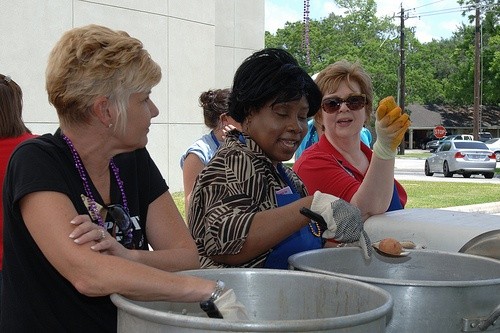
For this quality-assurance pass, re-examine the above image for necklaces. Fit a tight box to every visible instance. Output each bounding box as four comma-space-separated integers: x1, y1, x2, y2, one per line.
62, 133, 132, 250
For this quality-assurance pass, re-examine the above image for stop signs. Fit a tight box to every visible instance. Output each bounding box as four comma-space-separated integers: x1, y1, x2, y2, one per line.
432, 126, 447, 139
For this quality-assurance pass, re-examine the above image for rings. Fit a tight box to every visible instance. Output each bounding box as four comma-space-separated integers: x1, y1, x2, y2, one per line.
100, 228, 106, 238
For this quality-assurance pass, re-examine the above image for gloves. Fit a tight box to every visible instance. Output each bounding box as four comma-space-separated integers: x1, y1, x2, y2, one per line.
200, 287, 250, 321
310, 191, 372, 262
372, 104, 411, 160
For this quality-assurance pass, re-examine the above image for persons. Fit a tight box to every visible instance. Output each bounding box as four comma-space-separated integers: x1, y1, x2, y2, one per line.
0, 72, 41, 299
0, 24, 250, 332
186, 48, 371, 270
291, 59, 412, 249
180, 87, 232, 224
294, 71, 372, 163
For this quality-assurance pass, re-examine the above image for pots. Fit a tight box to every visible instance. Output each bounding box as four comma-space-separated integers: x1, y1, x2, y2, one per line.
289, 244, 500, 333
111, 268, 393, 333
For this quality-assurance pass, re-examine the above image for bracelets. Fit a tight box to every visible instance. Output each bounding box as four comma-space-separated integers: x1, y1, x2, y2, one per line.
210, 279, 225, 301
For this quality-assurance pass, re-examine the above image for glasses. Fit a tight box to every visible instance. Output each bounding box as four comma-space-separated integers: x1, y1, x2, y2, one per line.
320, 95, 369, 114
103, 204, 133, 250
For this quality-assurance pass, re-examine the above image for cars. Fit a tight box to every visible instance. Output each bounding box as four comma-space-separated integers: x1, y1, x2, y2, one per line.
425, 134, 473, 150
477, 132, 492, 141
424, 140, 497, 179
483, 138, 500, 163
